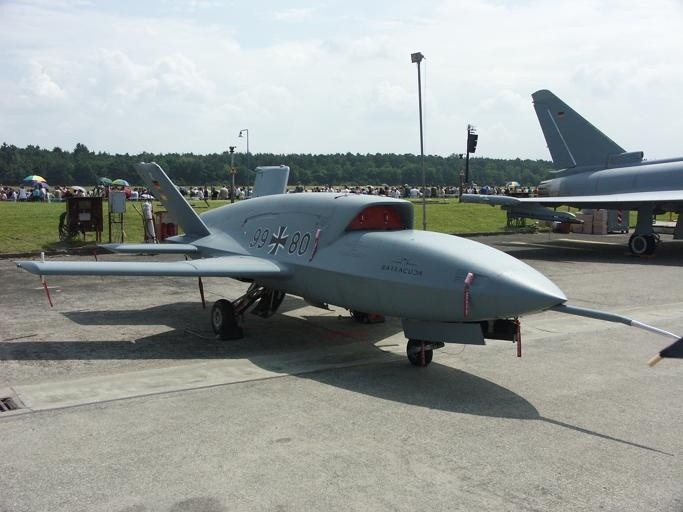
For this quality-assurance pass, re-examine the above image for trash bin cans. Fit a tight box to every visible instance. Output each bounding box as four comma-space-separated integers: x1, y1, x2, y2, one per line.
430, 187, 437, 197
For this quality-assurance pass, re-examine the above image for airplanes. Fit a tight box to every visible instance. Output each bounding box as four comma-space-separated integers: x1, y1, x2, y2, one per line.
460, 89, 683, 254
12, 160, 683, 367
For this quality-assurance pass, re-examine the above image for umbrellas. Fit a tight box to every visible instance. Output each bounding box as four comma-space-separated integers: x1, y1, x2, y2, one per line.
31, 182, 49, 187
506, 181, 520, 188
22, 175, 47, 183
97, 177, 112, 185
112, 179, 130, 187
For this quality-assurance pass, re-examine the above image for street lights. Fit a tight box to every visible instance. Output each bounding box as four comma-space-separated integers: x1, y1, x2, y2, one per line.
229, 146, 234, 201
411, 53, 427, 228
239, 129, 250, 196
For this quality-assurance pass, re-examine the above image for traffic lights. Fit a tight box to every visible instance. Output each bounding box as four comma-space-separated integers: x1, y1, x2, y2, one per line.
468, 133, 478, 153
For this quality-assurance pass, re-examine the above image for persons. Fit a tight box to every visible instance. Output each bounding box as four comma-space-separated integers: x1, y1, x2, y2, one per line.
175, 185, 252, 200
0, 184, 158, 202
285, 183, 539, 199
